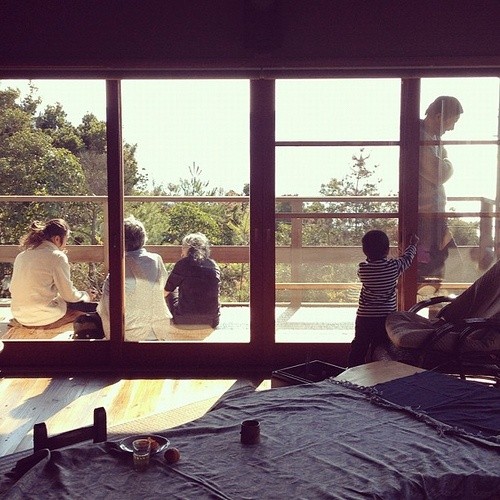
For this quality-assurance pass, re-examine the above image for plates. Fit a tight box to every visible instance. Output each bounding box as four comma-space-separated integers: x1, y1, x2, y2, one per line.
120, 435, 169, 454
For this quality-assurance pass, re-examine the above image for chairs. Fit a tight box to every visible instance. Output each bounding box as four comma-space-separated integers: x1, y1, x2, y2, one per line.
365, 259, 500, 376
33, 407, 107, 454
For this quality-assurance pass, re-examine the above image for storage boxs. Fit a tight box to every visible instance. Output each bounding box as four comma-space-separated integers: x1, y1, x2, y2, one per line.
271, 360, 346, 388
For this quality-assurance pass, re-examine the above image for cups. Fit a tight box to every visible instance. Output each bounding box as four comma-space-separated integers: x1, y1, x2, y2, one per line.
241, 419, 261, 445
133, 440, 150, 469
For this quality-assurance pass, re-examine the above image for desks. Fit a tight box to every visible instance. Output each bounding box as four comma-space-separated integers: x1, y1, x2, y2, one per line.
0, 360, 500, 500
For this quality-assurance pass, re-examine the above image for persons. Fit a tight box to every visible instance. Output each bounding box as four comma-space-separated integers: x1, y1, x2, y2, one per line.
96, 214, 173, 341
9, 219, 90, 329
347, 230, 420, 369
164, 233, 221, 330
417, 96, 463, 319
